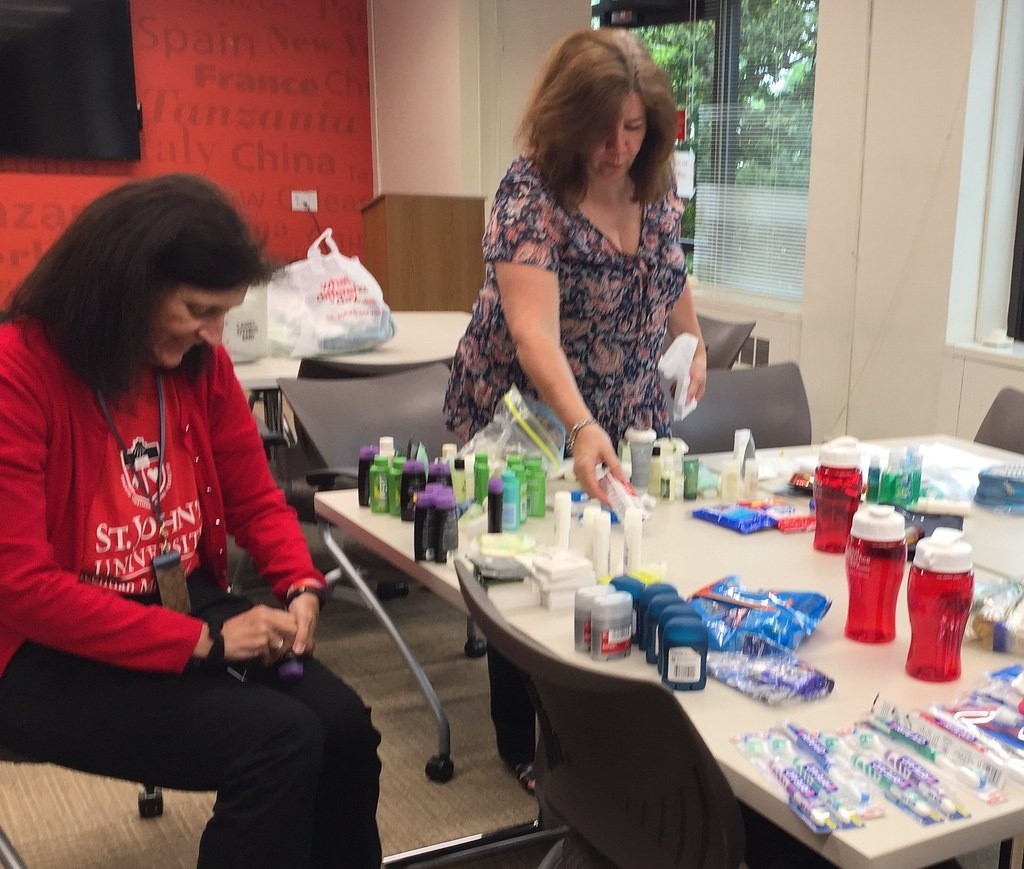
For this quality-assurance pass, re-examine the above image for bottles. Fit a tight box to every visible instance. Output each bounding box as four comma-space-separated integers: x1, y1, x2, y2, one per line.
844, 502, 907, 646
908, 528, 974, 683
814, 436, 862, 553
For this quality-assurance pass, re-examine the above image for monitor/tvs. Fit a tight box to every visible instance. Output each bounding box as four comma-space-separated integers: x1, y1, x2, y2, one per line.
0, 0, 142, 162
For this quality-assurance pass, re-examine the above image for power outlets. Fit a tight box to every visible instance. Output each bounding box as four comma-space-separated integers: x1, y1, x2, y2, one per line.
291, 190, 317, 212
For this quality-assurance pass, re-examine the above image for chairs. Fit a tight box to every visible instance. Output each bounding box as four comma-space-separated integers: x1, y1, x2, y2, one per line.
973, 387, 1024, 454
226, 356, 438, 608
660, 361, 812, 456
277, 361, 489, 783
453, 558, 962, 867
661, 312, 758, 372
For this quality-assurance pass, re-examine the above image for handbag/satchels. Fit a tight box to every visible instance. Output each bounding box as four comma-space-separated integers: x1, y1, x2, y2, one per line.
223, 228, 395, 364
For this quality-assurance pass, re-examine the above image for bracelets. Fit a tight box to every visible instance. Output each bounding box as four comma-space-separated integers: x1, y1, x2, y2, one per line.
284, 575, 327, 610
201, 619, 226, 673
564, 415, 600, 451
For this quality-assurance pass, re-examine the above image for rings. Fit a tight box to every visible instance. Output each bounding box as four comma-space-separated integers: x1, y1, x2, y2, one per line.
270, 634, 284, 653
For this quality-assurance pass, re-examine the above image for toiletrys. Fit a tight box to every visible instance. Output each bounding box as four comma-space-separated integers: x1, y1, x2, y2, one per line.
901, 527, 980, 682
611, 576, 643, 645
659, 616, 707, 692
589, 589, 634, 663
637, 582, 678, 652
353, 431, 922, 613
813, 435, 863, 554
643, 593, 685, 666
842, 502, 908, 645
657, 603, 703, 675
572, 584, 616, 653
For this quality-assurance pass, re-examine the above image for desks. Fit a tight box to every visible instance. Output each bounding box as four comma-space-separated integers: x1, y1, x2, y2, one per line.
313, 432, 1024, 869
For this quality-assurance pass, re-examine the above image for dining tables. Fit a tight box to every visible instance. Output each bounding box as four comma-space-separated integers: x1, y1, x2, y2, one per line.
298, 311, 475, 376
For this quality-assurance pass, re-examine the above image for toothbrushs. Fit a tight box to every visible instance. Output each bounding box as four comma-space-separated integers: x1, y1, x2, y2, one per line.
729, 689, 1020, 836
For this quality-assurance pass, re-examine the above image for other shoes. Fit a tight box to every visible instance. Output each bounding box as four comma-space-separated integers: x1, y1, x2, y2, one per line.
516, 763, 535, 793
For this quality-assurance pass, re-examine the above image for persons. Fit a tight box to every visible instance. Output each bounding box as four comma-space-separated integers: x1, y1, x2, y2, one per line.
443, 25, 708, 793
0, 170, 382, 869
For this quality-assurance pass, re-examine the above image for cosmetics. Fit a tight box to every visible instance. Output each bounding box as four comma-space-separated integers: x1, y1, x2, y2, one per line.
351, 425, 928, 589
